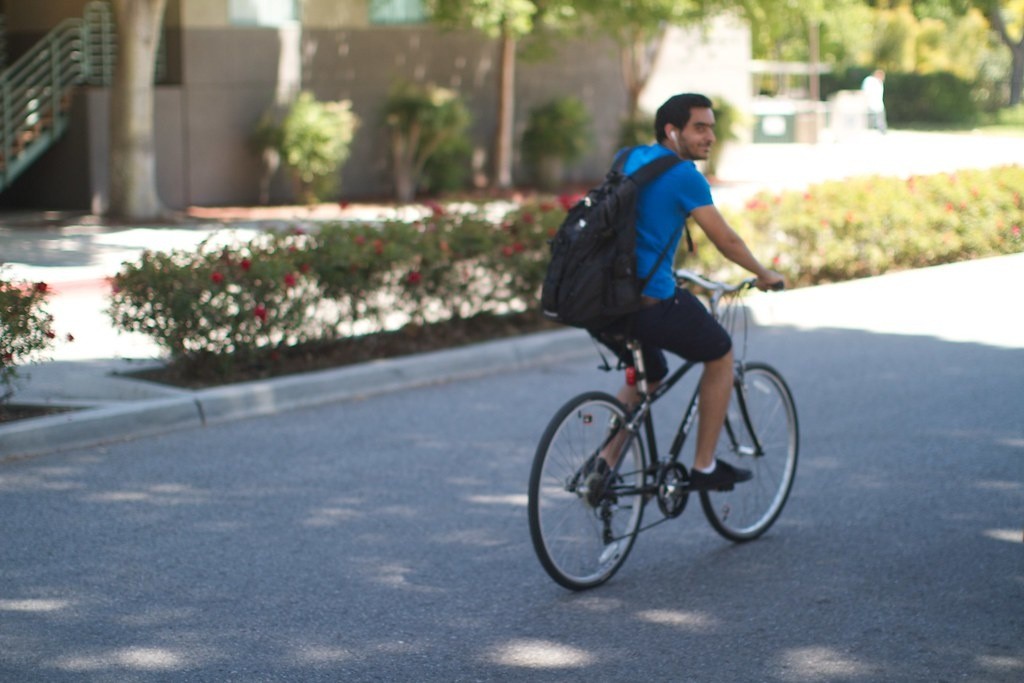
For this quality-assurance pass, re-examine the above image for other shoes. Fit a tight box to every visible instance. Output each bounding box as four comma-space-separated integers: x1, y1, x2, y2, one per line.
689, 458, 752, 489
594, 461, 618, 504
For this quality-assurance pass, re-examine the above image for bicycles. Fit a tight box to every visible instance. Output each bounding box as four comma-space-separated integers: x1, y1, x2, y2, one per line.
525, 264, 803, 594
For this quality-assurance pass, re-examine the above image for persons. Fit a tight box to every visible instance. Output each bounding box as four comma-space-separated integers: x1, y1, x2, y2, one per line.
586, 93, 781, 505
861, 70, 888, 135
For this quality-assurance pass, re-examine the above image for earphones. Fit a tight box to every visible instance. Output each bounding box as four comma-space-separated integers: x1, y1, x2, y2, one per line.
670, 130, 676, 144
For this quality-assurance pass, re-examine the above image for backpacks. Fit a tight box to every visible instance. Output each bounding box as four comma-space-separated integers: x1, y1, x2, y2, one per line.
541, 146, 687, 325
